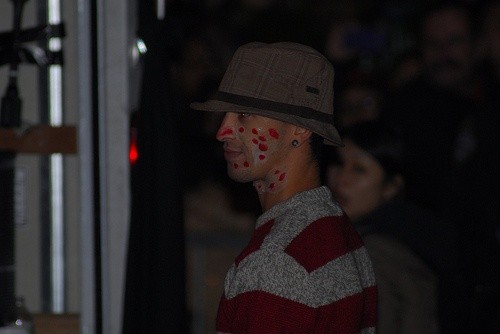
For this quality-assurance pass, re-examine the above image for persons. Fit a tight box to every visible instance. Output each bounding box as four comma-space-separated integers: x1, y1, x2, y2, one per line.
388, 0, 499, 216
327, 122, 444, 334
187, 43, 378, 333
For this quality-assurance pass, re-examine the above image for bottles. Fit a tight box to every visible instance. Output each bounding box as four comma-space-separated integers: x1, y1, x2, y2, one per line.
8, 298, 34, 334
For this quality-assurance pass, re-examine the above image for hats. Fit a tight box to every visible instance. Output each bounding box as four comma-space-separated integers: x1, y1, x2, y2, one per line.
196, 40, 347, 147
